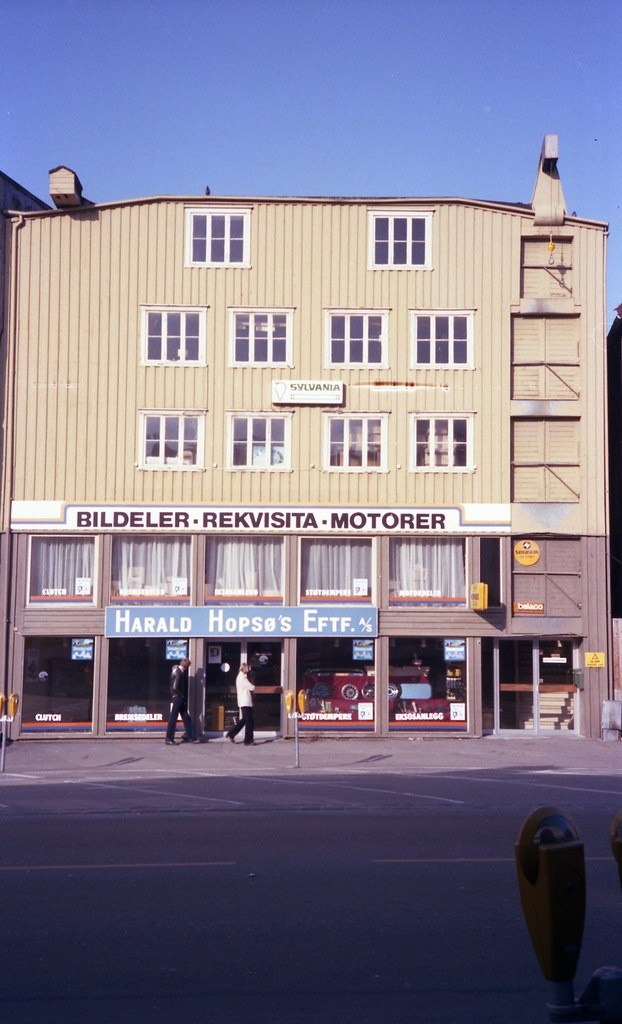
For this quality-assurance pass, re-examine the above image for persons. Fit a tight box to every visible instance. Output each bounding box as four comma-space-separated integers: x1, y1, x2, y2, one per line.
225, 663, 257, 746
165, 659, 201, 745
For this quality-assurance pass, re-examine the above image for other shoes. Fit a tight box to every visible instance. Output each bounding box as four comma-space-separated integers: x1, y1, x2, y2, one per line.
225, 734, 235, 744
166, 738, 177, 745
244, 741, 256, 746
190, 739, 200, 744
